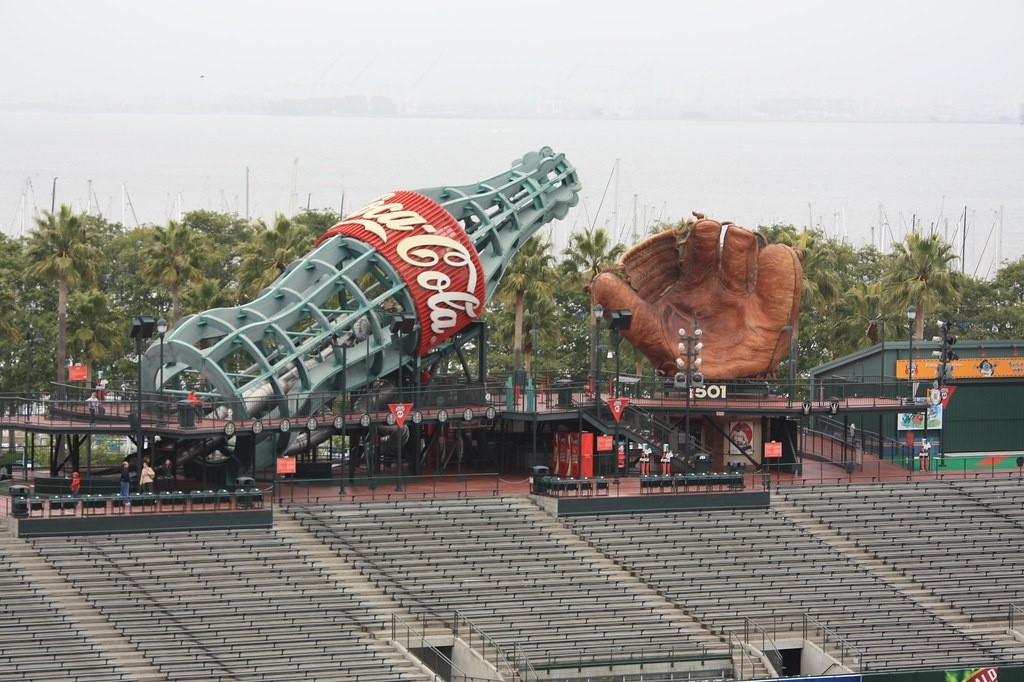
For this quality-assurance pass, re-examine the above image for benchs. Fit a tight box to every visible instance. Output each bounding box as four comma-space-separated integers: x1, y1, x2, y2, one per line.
0, 526, 414, 682
560, 506, 1024, 672
771, 476, 1024, 506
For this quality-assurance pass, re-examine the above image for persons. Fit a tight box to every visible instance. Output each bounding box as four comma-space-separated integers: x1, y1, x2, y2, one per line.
121, 456, 174, 505
70, 472, 81, 508
188, 390, 199, 416
85, 392, 100, 423
918, 433, 931, 472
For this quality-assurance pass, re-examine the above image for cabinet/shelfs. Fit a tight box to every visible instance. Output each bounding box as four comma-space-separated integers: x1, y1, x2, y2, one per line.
278, 489, 735, 681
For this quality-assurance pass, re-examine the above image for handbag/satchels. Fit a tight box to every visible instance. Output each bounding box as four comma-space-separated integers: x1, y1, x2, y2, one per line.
147, 467, 156, 478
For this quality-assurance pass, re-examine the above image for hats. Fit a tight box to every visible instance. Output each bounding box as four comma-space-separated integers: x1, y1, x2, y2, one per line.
73, 472, 78, 476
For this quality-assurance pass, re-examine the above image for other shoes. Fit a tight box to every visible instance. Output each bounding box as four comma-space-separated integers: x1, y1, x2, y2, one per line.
123, 501, 130, 505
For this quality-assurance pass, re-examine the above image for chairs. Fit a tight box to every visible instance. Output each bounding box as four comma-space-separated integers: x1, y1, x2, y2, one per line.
190, 490, 203, 512
639, 472, 747, 496
217, 489, 232, 512
159, 491, 173, 513
62, 494, 78, 516
30, 495, 45, 518
81, 494, 94, 516
235, 488, 249, 510
596, 476, 609, 496
172, 490, 188, 512
111, 493, 125, 514
540, 475, 593, 498
249, 488, 264, 509
129, 492, 143, 514
203, 489, 217, 511
142, 491, 157, 513
14, 497, 28, 518
49, 495, 63, 517
93, 494, 107, 515
